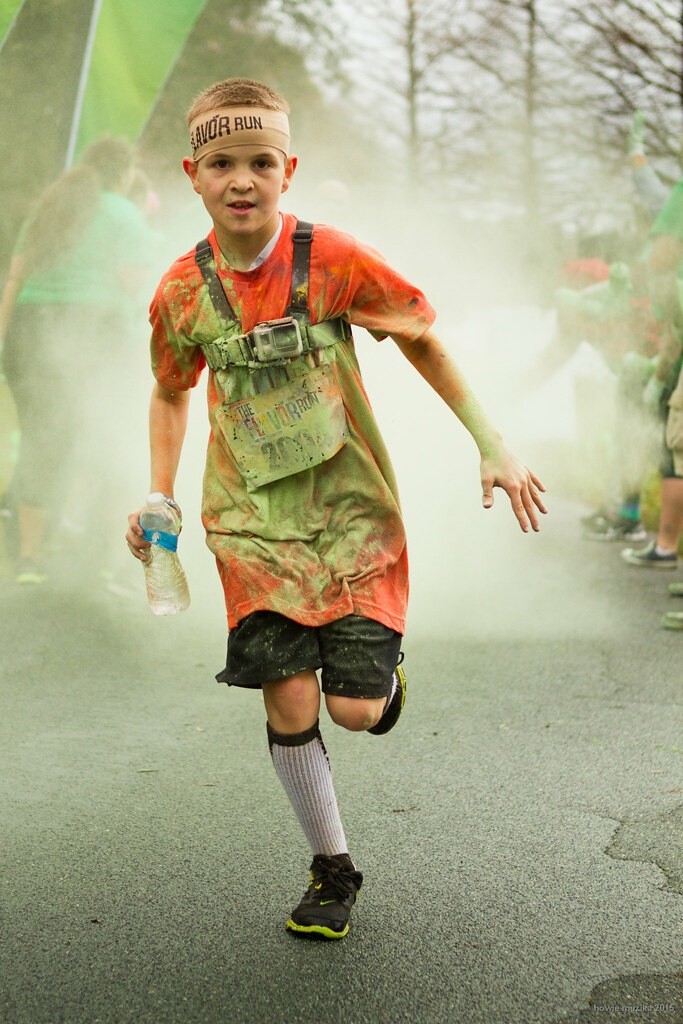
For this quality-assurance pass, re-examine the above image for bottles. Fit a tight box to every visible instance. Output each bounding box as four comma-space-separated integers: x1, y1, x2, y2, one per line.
138, 492, 191, 617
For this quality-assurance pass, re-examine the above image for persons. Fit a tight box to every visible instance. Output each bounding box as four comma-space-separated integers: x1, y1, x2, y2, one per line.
121, 78, 547, 940
0, 107, 683, 635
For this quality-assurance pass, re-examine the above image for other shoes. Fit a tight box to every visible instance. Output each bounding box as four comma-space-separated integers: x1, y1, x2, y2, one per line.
662, 610, 683, 628
588, 517, 647, 543
669, 583, 683, 595
14, 557, 47, 583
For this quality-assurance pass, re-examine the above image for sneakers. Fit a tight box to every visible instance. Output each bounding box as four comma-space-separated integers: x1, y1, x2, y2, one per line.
367, 663, 407, 736
285, 853, 364, 938
621, 540, 678, 568
